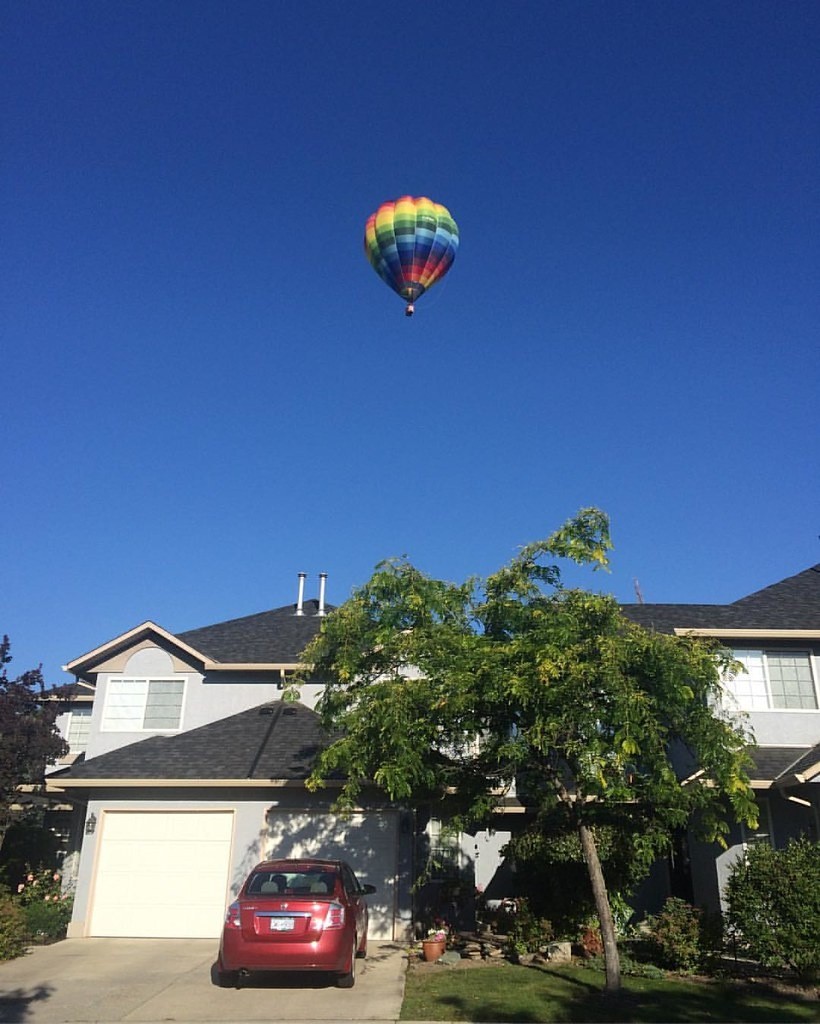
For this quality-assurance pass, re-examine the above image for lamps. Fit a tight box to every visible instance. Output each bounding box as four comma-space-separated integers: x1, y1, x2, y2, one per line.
86, 812, 96, 835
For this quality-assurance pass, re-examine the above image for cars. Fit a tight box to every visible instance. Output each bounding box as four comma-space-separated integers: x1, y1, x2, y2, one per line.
217, 856, 378, 988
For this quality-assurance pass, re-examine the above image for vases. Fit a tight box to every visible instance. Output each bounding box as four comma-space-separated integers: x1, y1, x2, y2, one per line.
422, 940, 445, 961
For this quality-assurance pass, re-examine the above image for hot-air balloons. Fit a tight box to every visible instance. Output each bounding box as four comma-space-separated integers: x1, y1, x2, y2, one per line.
364, 195, 461, 316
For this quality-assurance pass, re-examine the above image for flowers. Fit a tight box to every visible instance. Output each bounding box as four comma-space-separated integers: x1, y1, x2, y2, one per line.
426, 918, 447, 941
